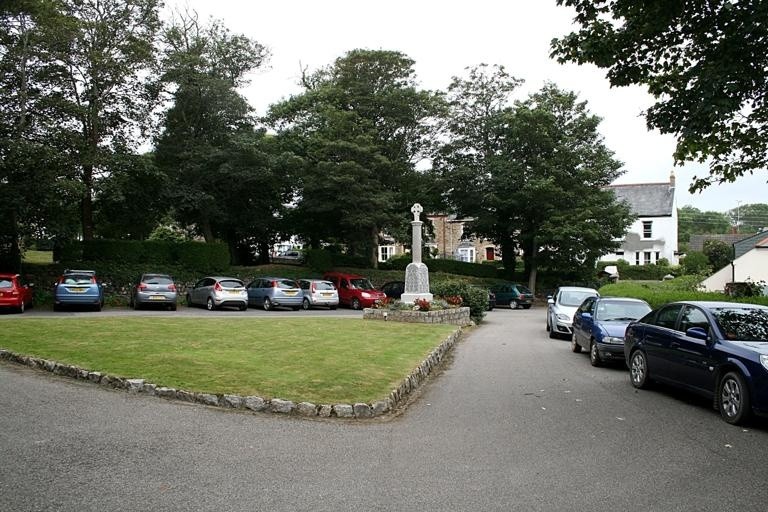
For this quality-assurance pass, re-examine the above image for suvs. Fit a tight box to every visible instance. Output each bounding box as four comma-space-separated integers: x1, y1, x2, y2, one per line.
322, 271, 387, 310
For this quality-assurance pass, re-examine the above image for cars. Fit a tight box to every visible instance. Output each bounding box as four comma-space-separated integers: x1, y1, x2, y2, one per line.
545, 285, 601, 340
186, 276, 248, 312
0, 273, 35, 313
130, 273, 178, 311
53, 267, 104, 312
624, 301, 768, 426
380, 281, 405, 299
246, 276, 340, 311
467, 283, 535, 311
275, 250, 305, 263
571, 296, 653, 368
